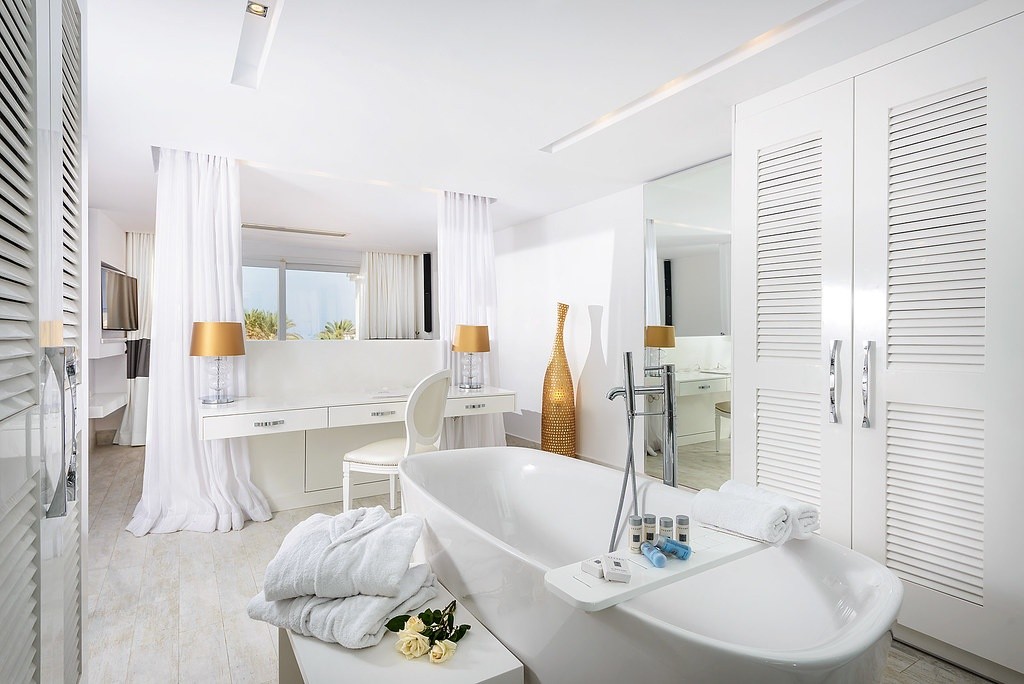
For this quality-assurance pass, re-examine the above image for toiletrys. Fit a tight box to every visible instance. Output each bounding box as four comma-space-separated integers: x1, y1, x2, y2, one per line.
628, 514, 691, 568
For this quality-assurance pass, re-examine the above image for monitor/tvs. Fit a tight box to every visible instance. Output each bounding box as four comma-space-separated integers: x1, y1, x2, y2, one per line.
100, 267, 139, 331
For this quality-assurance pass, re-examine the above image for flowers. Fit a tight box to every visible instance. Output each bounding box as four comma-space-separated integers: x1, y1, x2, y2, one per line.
384, 600, 471, 663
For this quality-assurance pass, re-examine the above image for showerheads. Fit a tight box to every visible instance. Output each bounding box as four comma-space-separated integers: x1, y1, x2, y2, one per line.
622, 351, 637, 419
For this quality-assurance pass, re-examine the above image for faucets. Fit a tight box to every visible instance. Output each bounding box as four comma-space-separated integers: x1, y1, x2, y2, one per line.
605, 363, 676, 402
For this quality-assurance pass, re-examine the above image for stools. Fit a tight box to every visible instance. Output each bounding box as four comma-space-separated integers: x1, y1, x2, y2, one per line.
278, 563, 524, 684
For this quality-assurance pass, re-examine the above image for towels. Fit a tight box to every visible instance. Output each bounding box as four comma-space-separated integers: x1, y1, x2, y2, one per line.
690, 479, 821, 549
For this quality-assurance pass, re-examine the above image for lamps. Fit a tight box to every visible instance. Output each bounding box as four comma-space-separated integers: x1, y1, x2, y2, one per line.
190, 322, 246, 405
451, 324, 490, 389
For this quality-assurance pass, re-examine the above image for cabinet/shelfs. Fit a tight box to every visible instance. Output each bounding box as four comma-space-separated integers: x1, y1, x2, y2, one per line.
730, 0, 1024, 684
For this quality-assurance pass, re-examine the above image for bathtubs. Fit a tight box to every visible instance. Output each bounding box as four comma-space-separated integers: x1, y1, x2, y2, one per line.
397, 444, 906, 683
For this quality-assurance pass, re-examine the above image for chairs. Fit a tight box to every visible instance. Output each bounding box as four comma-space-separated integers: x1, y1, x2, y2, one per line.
714, 401, 731, 453
343, 369, 452, 512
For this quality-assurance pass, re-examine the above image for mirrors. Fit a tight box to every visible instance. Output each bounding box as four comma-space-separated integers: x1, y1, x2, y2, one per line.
642, 153, 733, 493
101, 267, 139, 331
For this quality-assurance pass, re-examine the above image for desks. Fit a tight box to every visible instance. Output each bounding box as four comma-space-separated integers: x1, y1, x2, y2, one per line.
643, 366, 732, 456
197, 385, 517, 441
